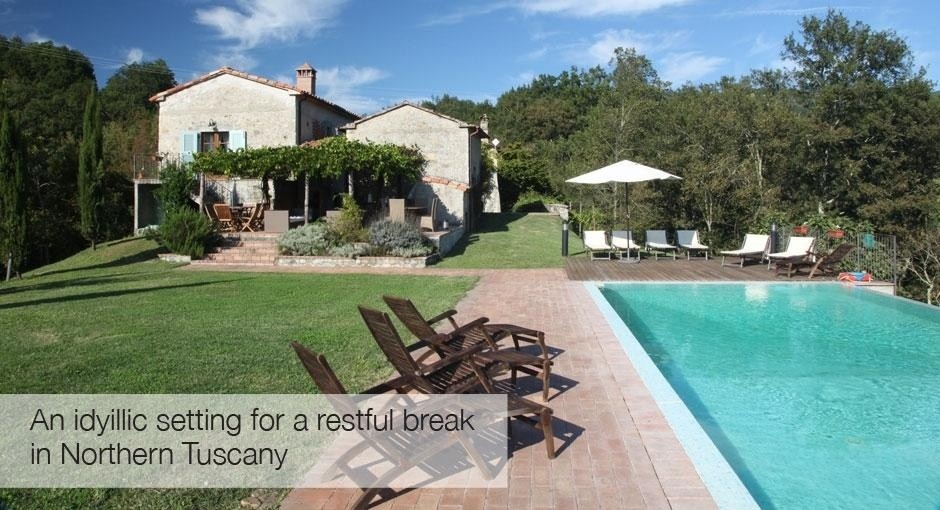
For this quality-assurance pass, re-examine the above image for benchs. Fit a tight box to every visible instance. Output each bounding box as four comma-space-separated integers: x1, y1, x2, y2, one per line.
644, 230, 676, 260
583, 231, 612, 261
805, 244, 855, 279
721, 234, 773, 269
612, 231, 640, 262
358, 304, 556, 405
383, 294, 548, 360
767, 236, 816, 278
676, 230, 709, 260
292, 340, 556, 510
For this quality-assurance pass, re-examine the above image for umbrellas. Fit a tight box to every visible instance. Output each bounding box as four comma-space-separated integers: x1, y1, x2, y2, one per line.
565, 160, 683, 259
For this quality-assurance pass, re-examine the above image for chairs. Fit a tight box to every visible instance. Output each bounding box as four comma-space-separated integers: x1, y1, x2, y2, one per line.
205, 201, 270, 232
